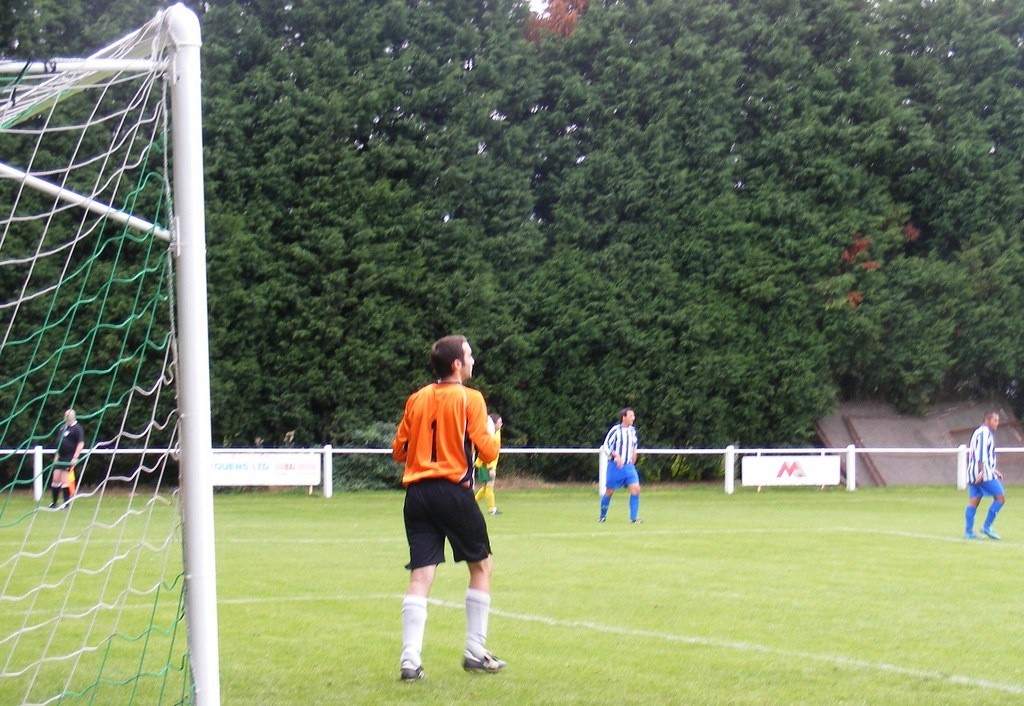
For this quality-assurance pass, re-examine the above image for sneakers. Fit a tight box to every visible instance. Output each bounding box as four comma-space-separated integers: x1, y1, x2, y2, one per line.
401, 661, 423, 682
463, 650, 506, 675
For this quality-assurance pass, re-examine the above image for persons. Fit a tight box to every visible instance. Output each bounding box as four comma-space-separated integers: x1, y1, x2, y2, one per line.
598, 407, 642, 524
48, 409, 86, 510
963, 411, 1005, 540
391, 335, 506, 679
472, 413, 504, 515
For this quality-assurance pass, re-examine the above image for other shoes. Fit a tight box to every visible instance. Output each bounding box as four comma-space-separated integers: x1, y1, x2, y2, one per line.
965, 532, 981, 539
599, 517, 606, 523
489, 509, 503, 514
629, 517, 642, 525
981, 526, 1000, 540
